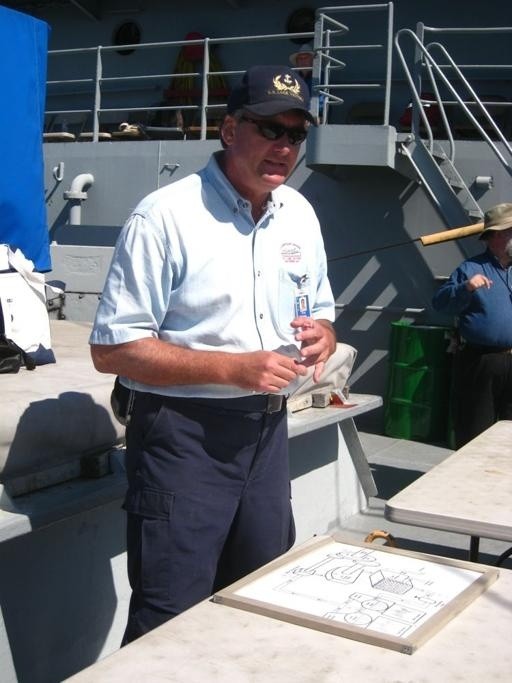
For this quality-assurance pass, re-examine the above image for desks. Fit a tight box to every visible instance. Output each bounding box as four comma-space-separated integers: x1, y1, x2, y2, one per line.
380, 420, 512, 535
52, 535, 512, 683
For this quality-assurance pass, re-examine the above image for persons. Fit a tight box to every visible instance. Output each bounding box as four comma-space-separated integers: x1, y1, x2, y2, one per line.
87, 62, 337, 649
431, 201, 512, 445
288, 41, 325, 126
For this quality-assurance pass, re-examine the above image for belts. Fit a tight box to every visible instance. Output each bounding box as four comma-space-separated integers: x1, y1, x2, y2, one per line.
190, 393, 288, 414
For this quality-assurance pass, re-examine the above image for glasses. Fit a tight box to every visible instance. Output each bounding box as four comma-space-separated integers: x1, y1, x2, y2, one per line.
237, 114, 308, 146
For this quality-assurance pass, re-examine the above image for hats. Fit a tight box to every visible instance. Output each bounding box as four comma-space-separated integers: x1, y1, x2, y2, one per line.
225, 64, 320, 130
477, 202, 512, 241
288, 44, 316, 67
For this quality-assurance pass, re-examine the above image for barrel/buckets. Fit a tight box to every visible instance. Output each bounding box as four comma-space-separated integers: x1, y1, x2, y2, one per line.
384, 326, 451, 440
